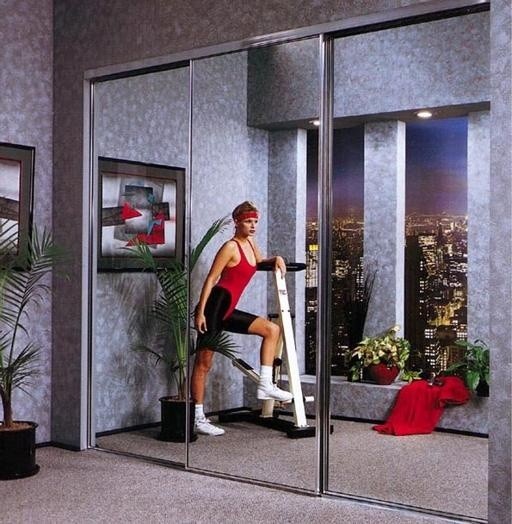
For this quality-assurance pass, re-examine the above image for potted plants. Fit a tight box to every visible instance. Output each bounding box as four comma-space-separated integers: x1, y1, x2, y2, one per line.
0, 211, 80, 480
113, 211, 272, 447
346, 321, 423, 387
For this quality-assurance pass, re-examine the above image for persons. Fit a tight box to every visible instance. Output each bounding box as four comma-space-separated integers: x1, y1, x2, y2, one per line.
189, 201, 294, 436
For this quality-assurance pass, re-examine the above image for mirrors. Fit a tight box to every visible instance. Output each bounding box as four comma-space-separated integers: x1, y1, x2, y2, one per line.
322, 6, 492, 524
186, 35, 321, 496
90, 64, 191, 476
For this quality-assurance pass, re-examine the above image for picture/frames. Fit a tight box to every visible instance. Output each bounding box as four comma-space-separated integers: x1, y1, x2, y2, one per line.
0, 141, 37, 273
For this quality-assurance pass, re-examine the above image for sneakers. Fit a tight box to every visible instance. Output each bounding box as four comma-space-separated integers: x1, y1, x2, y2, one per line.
193, 415, 225, 437
256, 382, 293, 402
